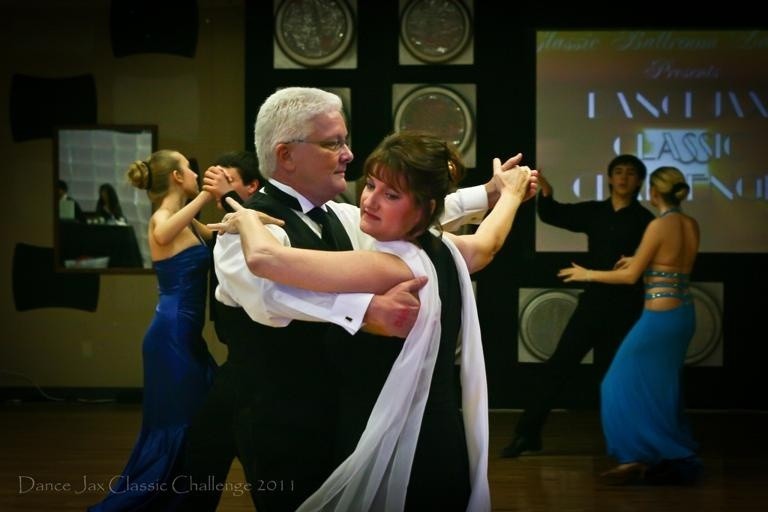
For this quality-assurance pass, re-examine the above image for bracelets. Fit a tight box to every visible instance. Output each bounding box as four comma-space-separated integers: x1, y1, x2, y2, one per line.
585, 268, 591, 282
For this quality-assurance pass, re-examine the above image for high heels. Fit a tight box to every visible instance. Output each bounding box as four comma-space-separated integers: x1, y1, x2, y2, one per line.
596, 458, 645, 482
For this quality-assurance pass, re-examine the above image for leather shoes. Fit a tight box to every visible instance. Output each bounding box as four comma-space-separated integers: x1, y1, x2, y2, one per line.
492, 432, 541, 459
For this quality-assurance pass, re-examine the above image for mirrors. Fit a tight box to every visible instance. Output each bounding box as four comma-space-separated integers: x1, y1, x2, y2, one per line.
49, 124, 160, 277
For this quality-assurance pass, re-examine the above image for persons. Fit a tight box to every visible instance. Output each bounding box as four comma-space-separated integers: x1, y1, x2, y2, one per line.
93, 182, 124, 223
204, 127, 531, 511
57, 179, 83, 219
499, 154, 657, 456
556, 166, 715, 488
127, 152, 264, 512
212, 82, 544, 512
85, 150, 233, 512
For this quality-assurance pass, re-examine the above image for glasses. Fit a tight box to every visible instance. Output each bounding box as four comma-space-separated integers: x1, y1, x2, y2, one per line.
286, 137, 350, 153
